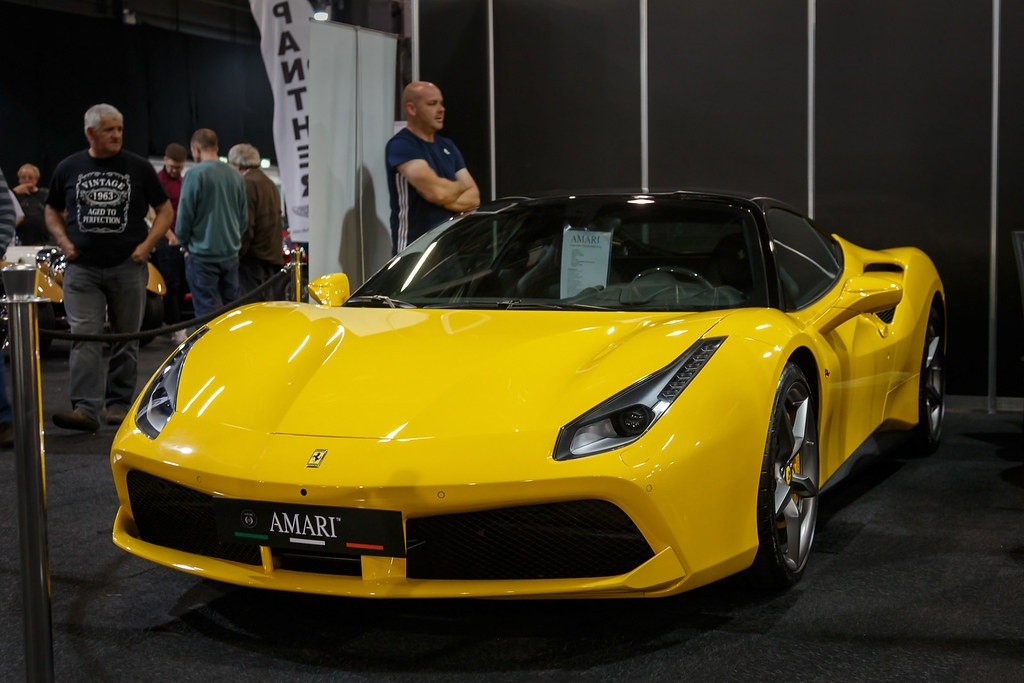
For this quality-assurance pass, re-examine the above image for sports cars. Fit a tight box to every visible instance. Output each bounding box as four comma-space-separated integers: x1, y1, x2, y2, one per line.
109, 189, 949, 599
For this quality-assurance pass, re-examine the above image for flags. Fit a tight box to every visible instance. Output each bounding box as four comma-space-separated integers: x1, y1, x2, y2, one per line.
249, 0, 317, 243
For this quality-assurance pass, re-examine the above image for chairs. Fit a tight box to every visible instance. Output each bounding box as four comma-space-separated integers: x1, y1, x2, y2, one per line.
702, 231, 800, 301
512, 226, 622, 298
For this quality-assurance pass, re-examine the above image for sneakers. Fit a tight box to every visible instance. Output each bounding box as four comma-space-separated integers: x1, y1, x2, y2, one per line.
105, 405, 128, 423
52, 408, 100, 432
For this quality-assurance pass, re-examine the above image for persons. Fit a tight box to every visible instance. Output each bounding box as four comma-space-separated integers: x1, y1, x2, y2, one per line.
229, 144, 284, 297
176, 129, 249, 321
44, 103, 174, 429
0, 168, 25, 267
12, 163, 50, 246
384, 81, 481, 255
150, 143, 187, 343
